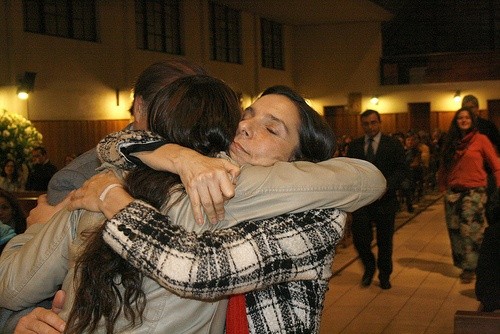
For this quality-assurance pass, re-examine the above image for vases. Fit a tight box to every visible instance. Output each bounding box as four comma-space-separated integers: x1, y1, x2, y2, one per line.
17, 162, 28, 189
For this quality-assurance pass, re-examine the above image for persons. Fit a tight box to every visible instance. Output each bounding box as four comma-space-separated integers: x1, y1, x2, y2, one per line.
345, 110, 407, 289
441, 95, 500, 313
389, 128, 448, 213
0, 60, 387, 334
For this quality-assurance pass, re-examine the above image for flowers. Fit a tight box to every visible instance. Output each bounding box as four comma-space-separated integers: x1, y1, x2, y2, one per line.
0, 108, 45, 174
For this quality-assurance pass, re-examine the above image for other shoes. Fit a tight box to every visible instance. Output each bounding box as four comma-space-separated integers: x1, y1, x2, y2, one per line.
361, 268, 376, 286
379, 276, 392, 290
460, 269, 475, 284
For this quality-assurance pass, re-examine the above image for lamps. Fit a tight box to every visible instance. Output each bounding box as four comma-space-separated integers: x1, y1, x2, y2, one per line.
370, 94, 379, 105
453, 89, 462, 101
17, 72, 38, 100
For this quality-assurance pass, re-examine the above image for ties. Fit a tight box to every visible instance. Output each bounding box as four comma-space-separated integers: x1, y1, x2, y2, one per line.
364, 139, 375, 162
